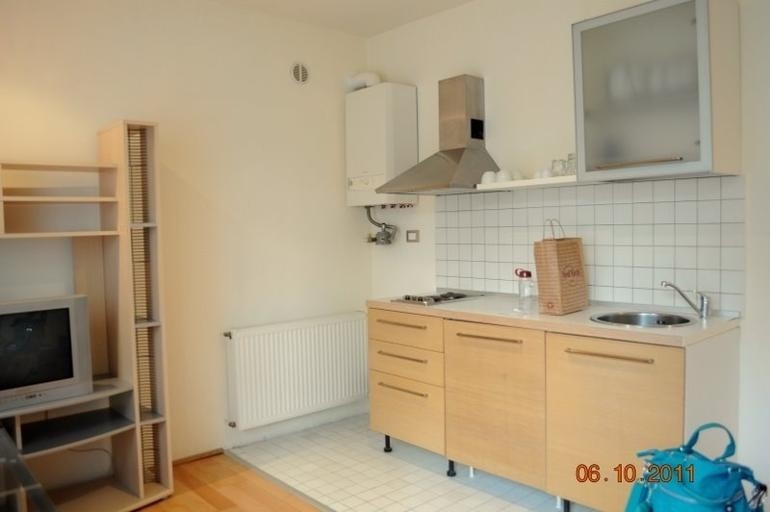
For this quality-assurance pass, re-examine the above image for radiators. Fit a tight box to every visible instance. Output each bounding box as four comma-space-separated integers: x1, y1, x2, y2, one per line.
225, 309, 367, 430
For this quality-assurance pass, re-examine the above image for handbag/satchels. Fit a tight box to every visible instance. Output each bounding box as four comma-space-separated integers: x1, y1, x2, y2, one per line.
624, 423, 767, 512
534, 219, 587, 315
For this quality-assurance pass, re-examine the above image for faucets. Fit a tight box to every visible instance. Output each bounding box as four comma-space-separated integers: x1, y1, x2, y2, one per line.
661, 280, 709, 321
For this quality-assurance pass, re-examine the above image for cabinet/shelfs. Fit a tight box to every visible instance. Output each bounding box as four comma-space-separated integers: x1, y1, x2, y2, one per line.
441, 307, 548, 495
572, 1, 739, 183
0, 120, 174, 512
548, 317, 740, 511
364, 302, 455, 476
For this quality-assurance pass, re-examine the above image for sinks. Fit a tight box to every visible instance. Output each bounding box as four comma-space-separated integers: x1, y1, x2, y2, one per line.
590, 312, 696, 330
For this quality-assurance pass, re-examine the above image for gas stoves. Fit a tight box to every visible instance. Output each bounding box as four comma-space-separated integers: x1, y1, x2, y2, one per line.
389, 290, 482, 306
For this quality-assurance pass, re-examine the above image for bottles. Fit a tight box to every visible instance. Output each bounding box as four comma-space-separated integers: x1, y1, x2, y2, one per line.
514, 267, 536, 313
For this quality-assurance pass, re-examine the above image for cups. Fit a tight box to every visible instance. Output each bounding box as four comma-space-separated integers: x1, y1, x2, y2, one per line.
476, 153, 577, 184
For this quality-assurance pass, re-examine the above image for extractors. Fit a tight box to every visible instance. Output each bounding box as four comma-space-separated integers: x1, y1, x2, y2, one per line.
375, 73, 513, 194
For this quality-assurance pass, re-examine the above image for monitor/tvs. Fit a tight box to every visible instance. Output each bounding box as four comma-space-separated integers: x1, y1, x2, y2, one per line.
0, 294, 94, 412
